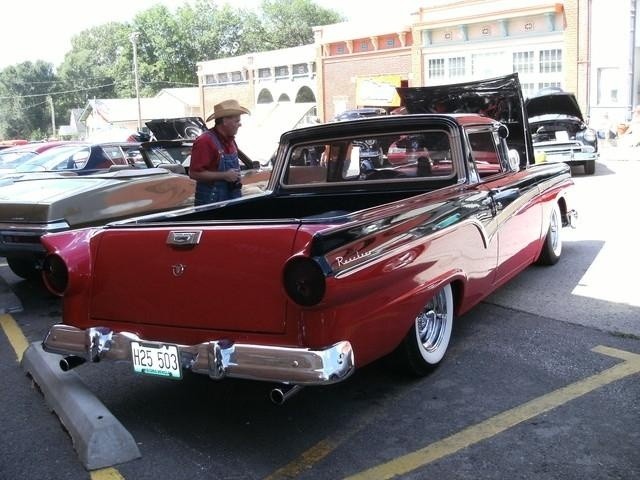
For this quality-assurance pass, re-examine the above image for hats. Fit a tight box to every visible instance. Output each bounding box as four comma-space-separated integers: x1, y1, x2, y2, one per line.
206, 101, 250, 122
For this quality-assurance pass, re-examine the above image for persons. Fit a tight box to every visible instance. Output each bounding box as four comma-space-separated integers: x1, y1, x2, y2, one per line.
307, 118, 325, 166
324, 119, 354, 177
188, 99, 251, 206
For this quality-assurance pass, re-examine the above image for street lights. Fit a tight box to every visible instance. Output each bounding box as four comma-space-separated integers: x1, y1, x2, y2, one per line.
127, 31, 142, 131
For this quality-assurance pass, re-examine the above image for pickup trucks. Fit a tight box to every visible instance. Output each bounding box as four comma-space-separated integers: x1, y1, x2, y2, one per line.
39, 70, 579, 407
